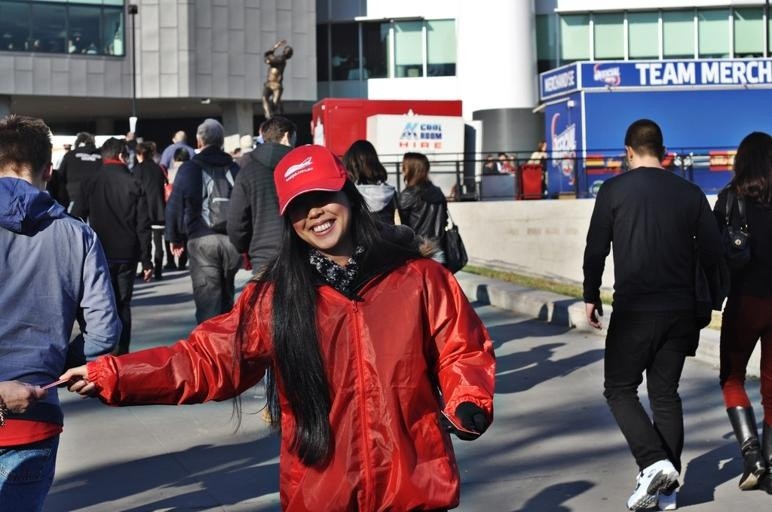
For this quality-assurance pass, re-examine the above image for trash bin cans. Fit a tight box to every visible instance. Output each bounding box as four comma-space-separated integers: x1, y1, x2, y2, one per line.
521, 164, 544, 200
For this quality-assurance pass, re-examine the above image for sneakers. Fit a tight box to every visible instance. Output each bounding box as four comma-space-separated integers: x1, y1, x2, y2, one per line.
626, 459, 679, 510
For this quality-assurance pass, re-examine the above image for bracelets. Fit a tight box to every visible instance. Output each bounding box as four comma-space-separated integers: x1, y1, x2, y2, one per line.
0, 396, 12, 427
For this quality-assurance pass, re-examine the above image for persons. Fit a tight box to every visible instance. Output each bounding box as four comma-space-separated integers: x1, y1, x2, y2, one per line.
57, 115, 296, 421
260, 44, 293, 121
340, 141, 400, 228
2, 31, 99, 56
483, 141, 551, 198
711, 131, 772, 492
0, 379, 50, 428
59, 144, 496, 512
397, 153, 450, 263
583, 118, 722, 510
0, 115, 122, 508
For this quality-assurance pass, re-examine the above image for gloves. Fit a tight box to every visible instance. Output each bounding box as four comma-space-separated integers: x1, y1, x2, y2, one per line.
438, 400, 489, 441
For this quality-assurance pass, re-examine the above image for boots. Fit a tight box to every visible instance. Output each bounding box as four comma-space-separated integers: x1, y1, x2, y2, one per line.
727, 405, 766, 489
758, 422, 772, 494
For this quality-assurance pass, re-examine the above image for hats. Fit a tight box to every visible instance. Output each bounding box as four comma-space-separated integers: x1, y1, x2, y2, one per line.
272, 144, 347, 216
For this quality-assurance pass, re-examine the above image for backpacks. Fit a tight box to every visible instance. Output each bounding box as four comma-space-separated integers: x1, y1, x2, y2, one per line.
193, 157, 236, 229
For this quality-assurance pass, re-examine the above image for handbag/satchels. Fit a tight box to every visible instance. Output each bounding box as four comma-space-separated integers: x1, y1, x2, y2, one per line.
164, 184, 173, 203
445, 224, 468, 273
724, 224, 752, 271
